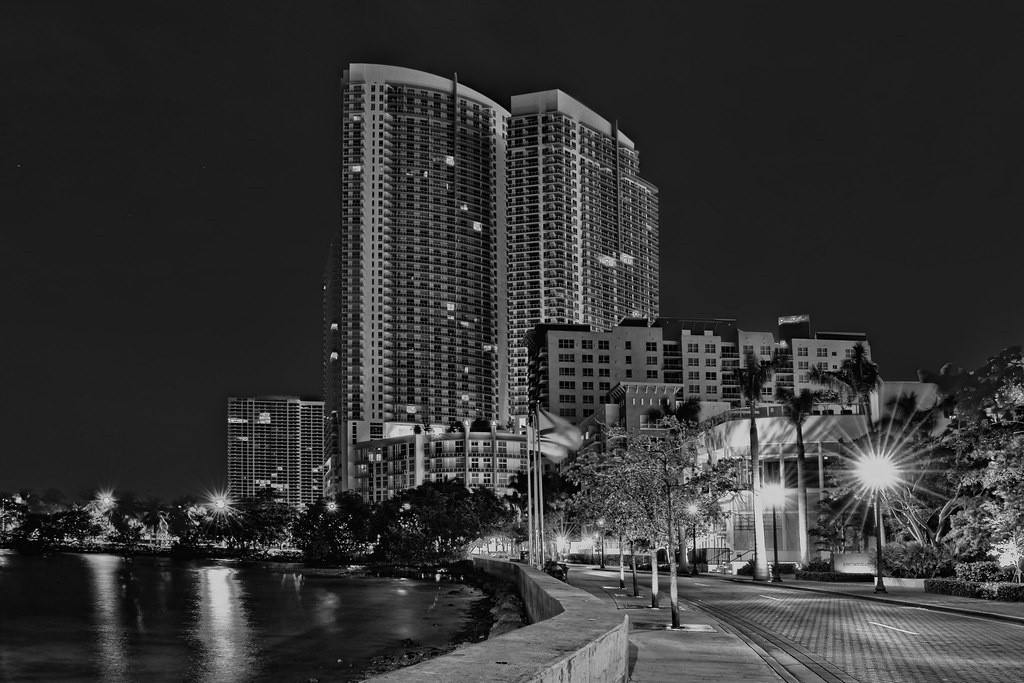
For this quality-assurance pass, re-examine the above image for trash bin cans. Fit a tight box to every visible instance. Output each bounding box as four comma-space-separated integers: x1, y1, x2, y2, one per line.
541, 560, 570, 584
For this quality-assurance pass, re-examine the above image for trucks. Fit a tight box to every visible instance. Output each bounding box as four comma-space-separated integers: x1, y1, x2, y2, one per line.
509, 551, 530, 564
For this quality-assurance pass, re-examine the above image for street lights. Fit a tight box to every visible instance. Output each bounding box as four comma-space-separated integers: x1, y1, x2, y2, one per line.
851, 449, 905, 595
597, 519, 607, 568
686, 505, 704, 577
759, 481, 792, 582
